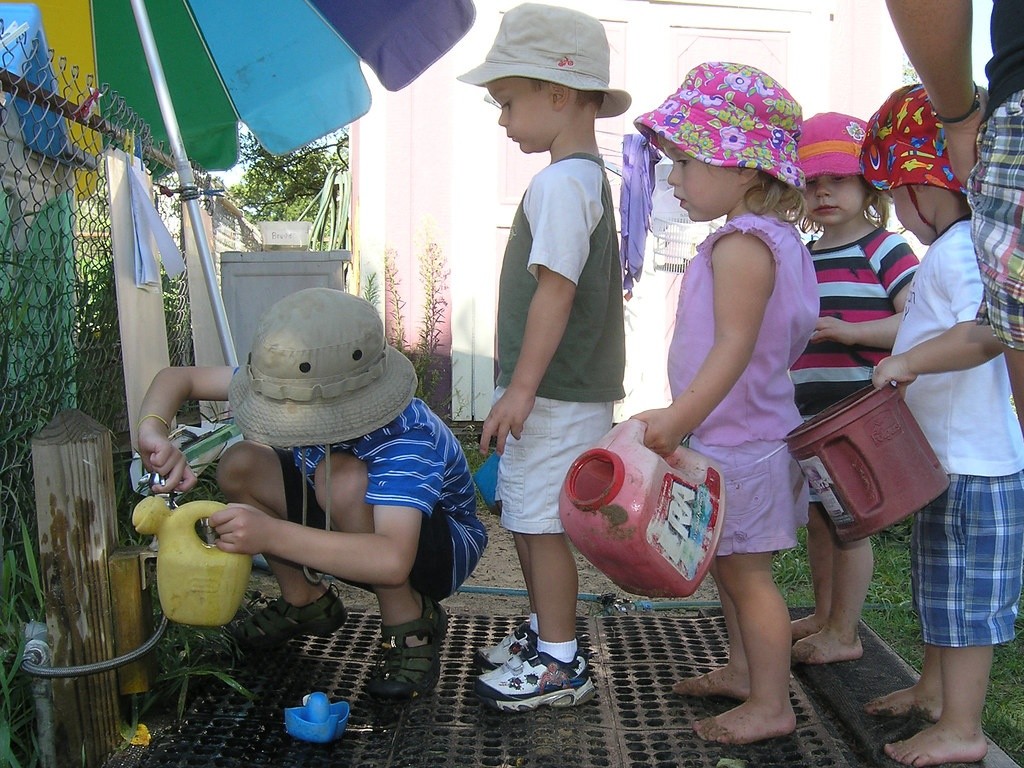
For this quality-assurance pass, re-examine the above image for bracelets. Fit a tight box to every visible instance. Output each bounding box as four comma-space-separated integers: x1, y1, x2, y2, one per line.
932, 80, 980, 123
138, 414, 171, 432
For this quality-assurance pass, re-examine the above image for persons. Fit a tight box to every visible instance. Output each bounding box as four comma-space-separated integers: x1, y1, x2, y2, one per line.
798, 112, 920, 663
458, 1, 632, 712
137, 288, 488, 698
629, 60, 821, 742
860, 0, 1024, 768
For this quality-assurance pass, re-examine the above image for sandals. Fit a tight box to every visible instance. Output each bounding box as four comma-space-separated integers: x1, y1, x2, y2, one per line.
366, 593, 449, 701
235, 586, 349, 653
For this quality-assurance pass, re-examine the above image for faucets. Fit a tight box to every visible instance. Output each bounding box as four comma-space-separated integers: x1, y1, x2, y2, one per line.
135, 468, 218, 551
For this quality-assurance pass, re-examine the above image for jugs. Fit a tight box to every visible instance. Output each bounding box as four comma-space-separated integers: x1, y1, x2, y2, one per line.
133, 496, 253, 627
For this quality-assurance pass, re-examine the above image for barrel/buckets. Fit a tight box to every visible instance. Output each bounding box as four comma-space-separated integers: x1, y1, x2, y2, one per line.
784, 380, 950, 542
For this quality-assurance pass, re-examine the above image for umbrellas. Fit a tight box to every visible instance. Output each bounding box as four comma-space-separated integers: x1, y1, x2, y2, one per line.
0, 0, 475, 367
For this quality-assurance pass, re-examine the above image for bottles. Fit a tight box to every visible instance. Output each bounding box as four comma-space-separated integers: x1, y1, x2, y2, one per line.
559, 420, 725, 599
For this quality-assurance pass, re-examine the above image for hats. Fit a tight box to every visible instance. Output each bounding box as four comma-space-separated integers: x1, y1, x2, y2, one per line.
226, 287, 418, 448
458, 2, 633, 118
630, 60, 806, 190
857, 83, 976, 194
789, 111, 872, 188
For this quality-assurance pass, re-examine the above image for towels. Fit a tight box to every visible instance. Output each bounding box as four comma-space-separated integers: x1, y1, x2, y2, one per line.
618, 132, 663, 293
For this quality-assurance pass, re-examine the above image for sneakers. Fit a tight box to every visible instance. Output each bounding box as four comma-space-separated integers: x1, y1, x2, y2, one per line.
475, 641, 597, 715
472, 621, 539, 675
